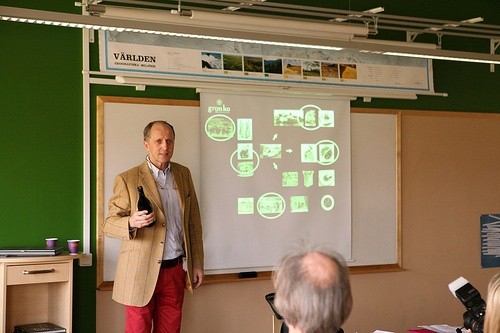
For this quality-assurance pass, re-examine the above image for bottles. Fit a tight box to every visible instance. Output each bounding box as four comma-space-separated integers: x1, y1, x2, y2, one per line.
136, 185, 156, 227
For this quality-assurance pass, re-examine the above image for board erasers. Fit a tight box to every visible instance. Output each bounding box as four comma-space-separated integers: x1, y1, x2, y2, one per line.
238, 271, 258, 278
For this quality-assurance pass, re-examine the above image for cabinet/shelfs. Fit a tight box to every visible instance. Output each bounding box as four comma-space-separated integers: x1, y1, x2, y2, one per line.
0, 251, 79, 333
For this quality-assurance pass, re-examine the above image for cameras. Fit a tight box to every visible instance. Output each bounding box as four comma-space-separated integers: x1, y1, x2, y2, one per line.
449, 276, 487, 333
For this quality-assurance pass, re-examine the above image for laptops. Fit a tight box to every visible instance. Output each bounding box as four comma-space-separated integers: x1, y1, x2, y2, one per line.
0, 246, 63, 256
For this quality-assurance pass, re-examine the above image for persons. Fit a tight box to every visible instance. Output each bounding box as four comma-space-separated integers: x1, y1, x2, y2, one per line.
102, 120, 206, 333
480, 271, 500, 333
271, 245, 353, 333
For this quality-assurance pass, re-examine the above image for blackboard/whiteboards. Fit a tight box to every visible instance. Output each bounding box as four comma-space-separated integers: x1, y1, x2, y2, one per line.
93, 93, 410, 292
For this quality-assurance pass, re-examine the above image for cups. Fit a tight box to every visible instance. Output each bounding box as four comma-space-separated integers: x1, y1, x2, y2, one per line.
45, 238, 59, 249
67, 239, 80, 255
